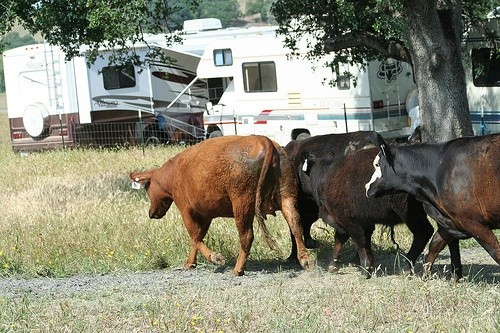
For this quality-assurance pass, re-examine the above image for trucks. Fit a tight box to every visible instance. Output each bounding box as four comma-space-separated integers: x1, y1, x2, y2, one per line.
197, 22, 419, 148
407, 7, 500, 142
2, 18, 294, 152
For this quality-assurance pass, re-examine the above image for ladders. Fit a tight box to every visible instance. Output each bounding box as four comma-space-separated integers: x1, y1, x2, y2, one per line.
384, 58, 401, 125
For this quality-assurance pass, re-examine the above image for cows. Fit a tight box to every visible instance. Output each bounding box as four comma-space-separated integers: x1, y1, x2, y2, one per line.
283, 125, 434, 280
129, 134, 315, 276
365, 125, 500, 283
